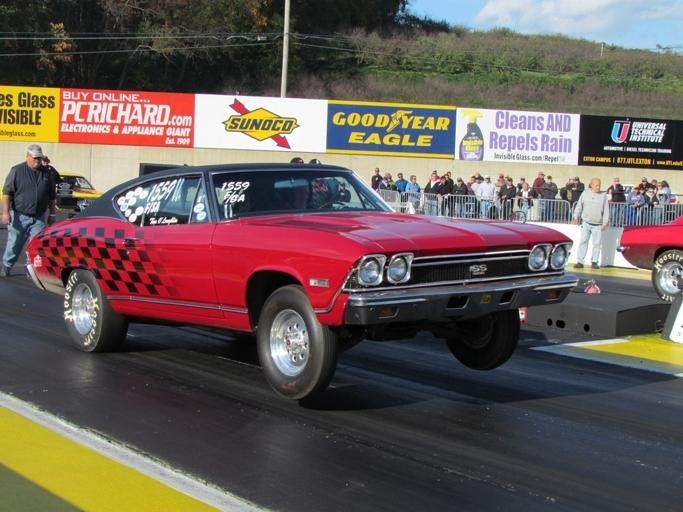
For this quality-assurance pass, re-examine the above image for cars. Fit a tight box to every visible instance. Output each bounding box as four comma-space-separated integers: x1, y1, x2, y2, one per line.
615, 215, 683, 302
601, 184, 678, 206
1, 171, 101, 211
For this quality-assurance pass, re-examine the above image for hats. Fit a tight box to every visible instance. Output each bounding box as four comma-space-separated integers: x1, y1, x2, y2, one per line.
27, 144, 43, 158
384, 170, 579, 182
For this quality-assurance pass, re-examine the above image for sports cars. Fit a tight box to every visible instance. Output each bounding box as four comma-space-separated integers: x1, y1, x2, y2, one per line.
22, 163, 580, 402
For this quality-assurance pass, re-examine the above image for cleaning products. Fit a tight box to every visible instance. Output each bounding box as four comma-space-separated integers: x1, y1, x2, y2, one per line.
458, 109, 485, 160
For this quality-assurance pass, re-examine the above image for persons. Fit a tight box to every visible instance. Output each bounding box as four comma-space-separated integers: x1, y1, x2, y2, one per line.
424, 172, 584, 222
608, 177, 672, 225
371, 169, 421, 214
0, 143, 57, 279
41, 154, 61, 225
570, 177, 610, 270
292, 158, 351, 208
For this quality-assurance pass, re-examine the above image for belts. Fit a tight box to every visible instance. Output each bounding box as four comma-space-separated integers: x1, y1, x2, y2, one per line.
585, 222, 600, 225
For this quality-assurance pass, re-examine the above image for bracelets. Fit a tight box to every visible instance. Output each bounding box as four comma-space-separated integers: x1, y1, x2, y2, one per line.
48, 213, 57, 218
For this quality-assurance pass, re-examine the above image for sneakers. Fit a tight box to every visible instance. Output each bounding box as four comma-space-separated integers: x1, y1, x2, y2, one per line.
574, 263, 584, 267
0, 265, 10, 278
592, 262, 599, 269
25, 273, 31, 279
